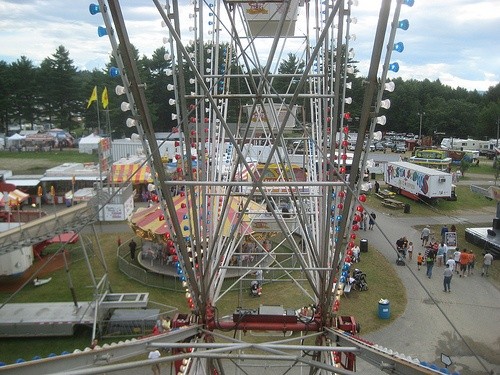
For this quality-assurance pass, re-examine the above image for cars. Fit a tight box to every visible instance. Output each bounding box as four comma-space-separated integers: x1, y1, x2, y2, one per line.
346, 139, 406, 154
24, 127, 75, 148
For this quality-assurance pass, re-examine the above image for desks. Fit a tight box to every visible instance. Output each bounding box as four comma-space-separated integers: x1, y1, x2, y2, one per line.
46, 196, 65, 203
384, 199, 403, 209
379, 191, 393, 198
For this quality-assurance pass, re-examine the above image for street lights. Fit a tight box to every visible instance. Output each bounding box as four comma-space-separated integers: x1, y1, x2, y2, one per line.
416, 111, 426, 146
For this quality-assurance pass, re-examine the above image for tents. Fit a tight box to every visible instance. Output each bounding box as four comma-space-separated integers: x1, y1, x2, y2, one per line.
79, 133, 103, 154
0, 189, 29, 207
8, 133, 25, 140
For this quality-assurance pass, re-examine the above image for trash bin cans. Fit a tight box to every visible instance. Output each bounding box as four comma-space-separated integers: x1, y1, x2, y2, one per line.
282, 205, 291, 218
404, 204, 410, 213
378, 301, 390, 319
266, 201, 275, 215
360, 238, 368, 252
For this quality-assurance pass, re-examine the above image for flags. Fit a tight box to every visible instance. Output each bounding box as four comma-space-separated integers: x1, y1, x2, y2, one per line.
86, 86, 97, 109
102, 87, 108, 109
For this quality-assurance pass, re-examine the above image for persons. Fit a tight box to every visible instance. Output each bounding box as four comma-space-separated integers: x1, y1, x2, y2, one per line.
456, 169, 461, 183
375, 181, 379, 193
396, 225, 493, 292
368, 182, 372, 191
129, 239, 137, 260
157, 315, 171, 333
148, 348, 161, 375
252, 269, 263, 284
359, 211, 376, 230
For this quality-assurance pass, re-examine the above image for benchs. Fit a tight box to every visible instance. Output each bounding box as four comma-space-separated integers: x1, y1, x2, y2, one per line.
382, 202, 405, 207
374, 192, 395, 198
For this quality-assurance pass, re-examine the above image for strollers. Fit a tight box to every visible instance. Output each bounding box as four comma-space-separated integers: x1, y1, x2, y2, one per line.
396, 246, 406, 266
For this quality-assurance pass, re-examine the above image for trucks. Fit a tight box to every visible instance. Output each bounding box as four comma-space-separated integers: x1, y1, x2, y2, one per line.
440, 137, 500, 157
